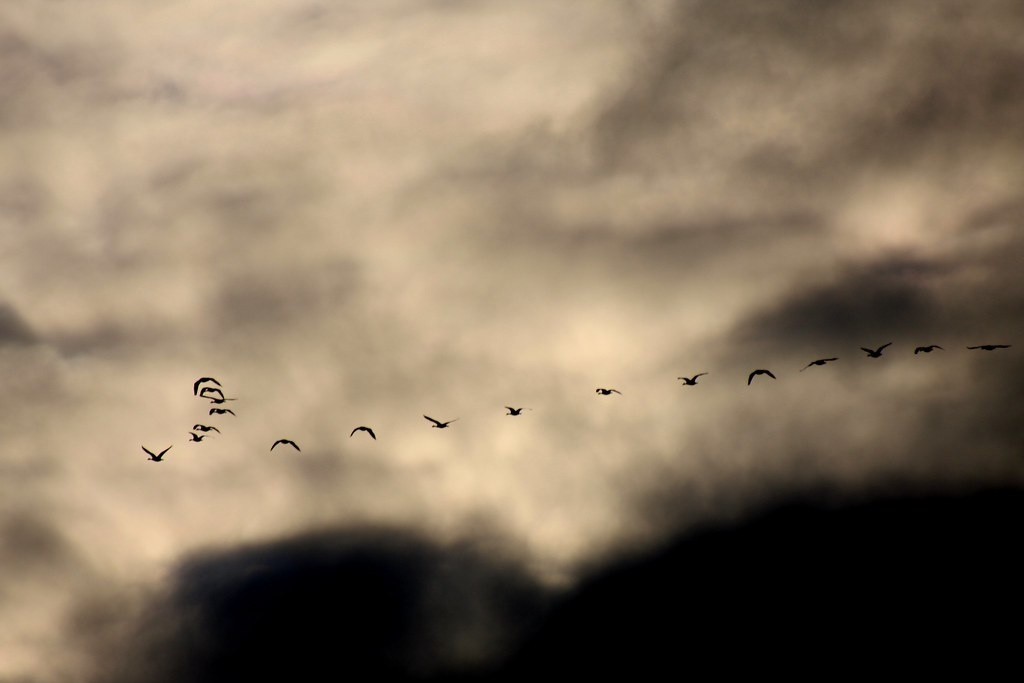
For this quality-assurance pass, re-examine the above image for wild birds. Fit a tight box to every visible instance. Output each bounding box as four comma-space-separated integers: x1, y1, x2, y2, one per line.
675, 372, 711, 386
269, 439, 303, 454
504, 404, 532, 417
422, 415, 458, 430
187, 376, 240, 442
746, 369, 777, 386
798, 357, 840, 373
859, 342, 893, 359
349, 426, 376, 441
965, 344, 1012, 352
913, 344, 945, 356
141, 444, 174, 463
595, 387, 625, 396
